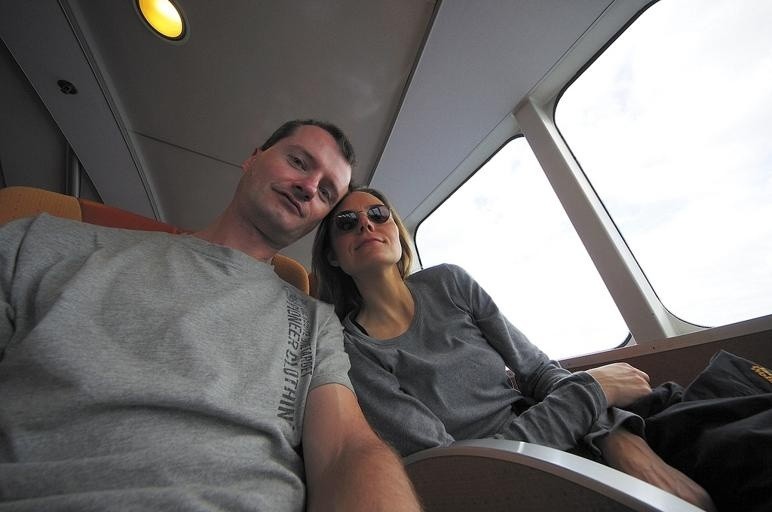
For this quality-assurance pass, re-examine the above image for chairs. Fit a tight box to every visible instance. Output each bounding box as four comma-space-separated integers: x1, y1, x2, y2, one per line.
1, 184, 309, 298
307, 270, 702, 511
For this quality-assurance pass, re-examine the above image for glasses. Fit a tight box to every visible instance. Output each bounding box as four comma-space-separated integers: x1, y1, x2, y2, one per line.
336, 205, 391, 231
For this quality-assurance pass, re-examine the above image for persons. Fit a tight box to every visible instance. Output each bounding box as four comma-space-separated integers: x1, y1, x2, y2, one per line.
312, 188, 772, 512
2, 119, 422, 510
